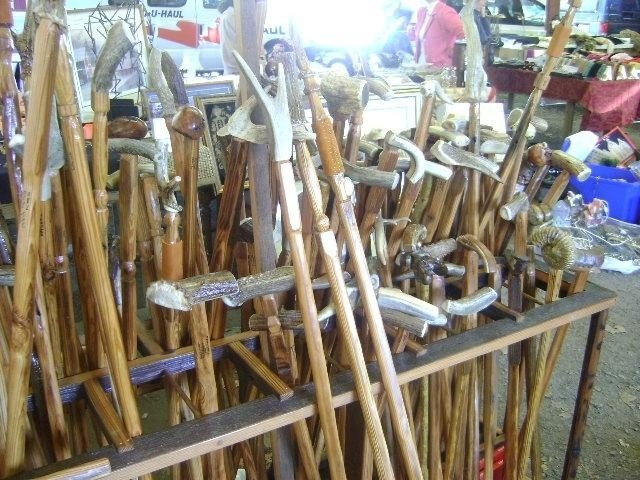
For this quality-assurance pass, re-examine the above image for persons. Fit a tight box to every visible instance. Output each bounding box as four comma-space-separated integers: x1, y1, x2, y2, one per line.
220, 0, 236, 76
473, 0, 492, 46
412, 1, 467, 88
263, 40, 294, 64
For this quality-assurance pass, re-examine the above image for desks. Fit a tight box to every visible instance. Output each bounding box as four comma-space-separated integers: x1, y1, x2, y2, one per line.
453, 65, 590, 139
